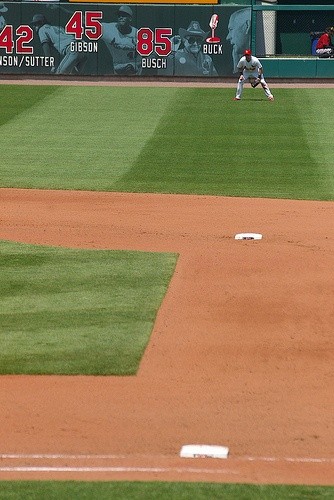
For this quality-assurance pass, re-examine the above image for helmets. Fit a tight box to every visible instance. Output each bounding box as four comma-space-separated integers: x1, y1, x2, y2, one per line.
244, 49, 251, 55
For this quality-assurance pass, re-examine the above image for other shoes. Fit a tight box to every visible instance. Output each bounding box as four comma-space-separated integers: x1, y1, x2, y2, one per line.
267, 95, 275, 101
233, 97, 242, 101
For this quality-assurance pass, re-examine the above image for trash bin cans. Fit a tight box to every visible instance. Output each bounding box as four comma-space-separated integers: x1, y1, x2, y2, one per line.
311, 32, 324, 56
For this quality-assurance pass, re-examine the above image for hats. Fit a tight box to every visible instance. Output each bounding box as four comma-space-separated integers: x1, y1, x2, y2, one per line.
30, 14, 47, 24
178, 21, 210, 41
116, 5, 133, 17
0, 2, 8, 13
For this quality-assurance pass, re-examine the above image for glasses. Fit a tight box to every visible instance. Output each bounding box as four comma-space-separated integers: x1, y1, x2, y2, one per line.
184, 37, 204, 46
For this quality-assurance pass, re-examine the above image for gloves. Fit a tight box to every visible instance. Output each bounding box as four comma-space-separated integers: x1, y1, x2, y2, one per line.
51, 67, 56, 73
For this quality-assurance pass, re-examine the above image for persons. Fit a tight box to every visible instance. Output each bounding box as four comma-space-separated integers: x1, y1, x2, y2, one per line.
165, 21, 217, 76
233, 49, 275, 102
315, 26, 334, 59
226, 6, 253, 74
46, 4, 147, 76
29, 14, 94, 76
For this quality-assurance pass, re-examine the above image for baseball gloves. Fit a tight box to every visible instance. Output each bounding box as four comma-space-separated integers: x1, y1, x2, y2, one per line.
251, 78, 260, 88
